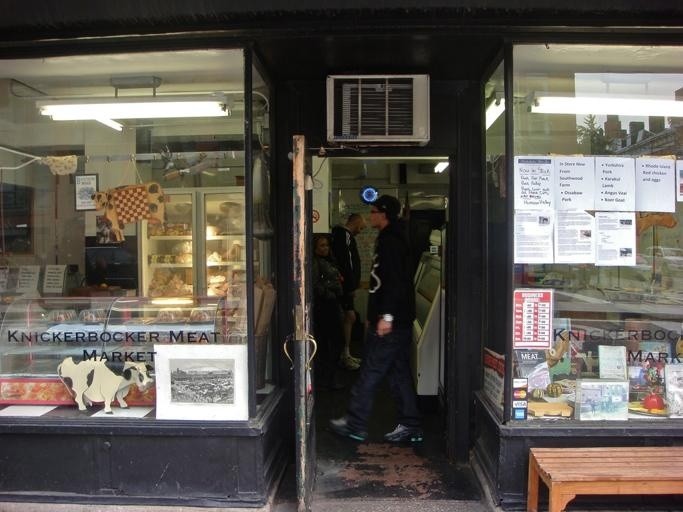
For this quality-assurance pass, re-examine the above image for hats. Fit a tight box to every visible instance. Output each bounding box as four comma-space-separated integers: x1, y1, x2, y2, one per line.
370, 195, 401, 215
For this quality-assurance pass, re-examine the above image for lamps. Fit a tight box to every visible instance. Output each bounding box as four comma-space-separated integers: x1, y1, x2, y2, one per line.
33, 74, 231, 123
523, 91, 681, 118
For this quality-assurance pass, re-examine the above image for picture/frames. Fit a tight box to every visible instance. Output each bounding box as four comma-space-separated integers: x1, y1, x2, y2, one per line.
73, 173, 98, 212
150, 342, 248, 422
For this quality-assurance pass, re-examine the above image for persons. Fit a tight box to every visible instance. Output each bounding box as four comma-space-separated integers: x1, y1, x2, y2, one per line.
309, 194, 425, 442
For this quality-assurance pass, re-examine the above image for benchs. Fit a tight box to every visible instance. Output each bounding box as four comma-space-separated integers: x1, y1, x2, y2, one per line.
527, 445, 683, 511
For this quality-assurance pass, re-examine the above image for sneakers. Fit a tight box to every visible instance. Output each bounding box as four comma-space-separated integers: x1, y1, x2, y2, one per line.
319, 382, 345, 393
384, 424, 423, 443
328, 417, 368, 441
338, 353, 363, 370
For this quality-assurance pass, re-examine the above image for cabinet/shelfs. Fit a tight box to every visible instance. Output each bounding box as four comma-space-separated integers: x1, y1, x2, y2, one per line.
0, 293, 225, 406
133, 185, 245, 318
